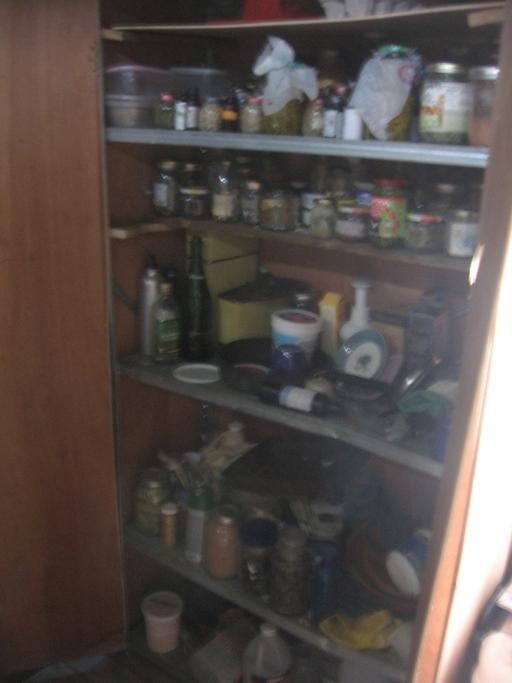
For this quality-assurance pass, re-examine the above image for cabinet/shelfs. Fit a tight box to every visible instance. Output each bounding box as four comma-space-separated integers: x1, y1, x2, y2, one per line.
95, 0, 512, 680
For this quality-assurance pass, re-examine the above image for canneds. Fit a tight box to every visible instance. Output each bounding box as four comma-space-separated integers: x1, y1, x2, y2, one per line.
205, 504, 277, 599
152, 62, 499, 261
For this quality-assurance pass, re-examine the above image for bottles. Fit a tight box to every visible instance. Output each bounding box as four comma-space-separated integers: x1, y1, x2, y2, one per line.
132, 473, 175, 537
418, 63, 500, 147
155, 85, 263, 134
180, 234, 218, 362
368, 177, 412, 250
204, 503, 243, 581
184, 486, 214, 564
405, 182, 481, 257
136, 254, 180, 364
152, 155, 370, 244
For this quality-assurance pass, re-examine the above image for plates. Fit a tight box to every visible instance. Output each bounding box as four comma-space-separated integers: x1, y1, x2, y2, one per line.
332, 328, 390, 380
172, 363, 224, 386
